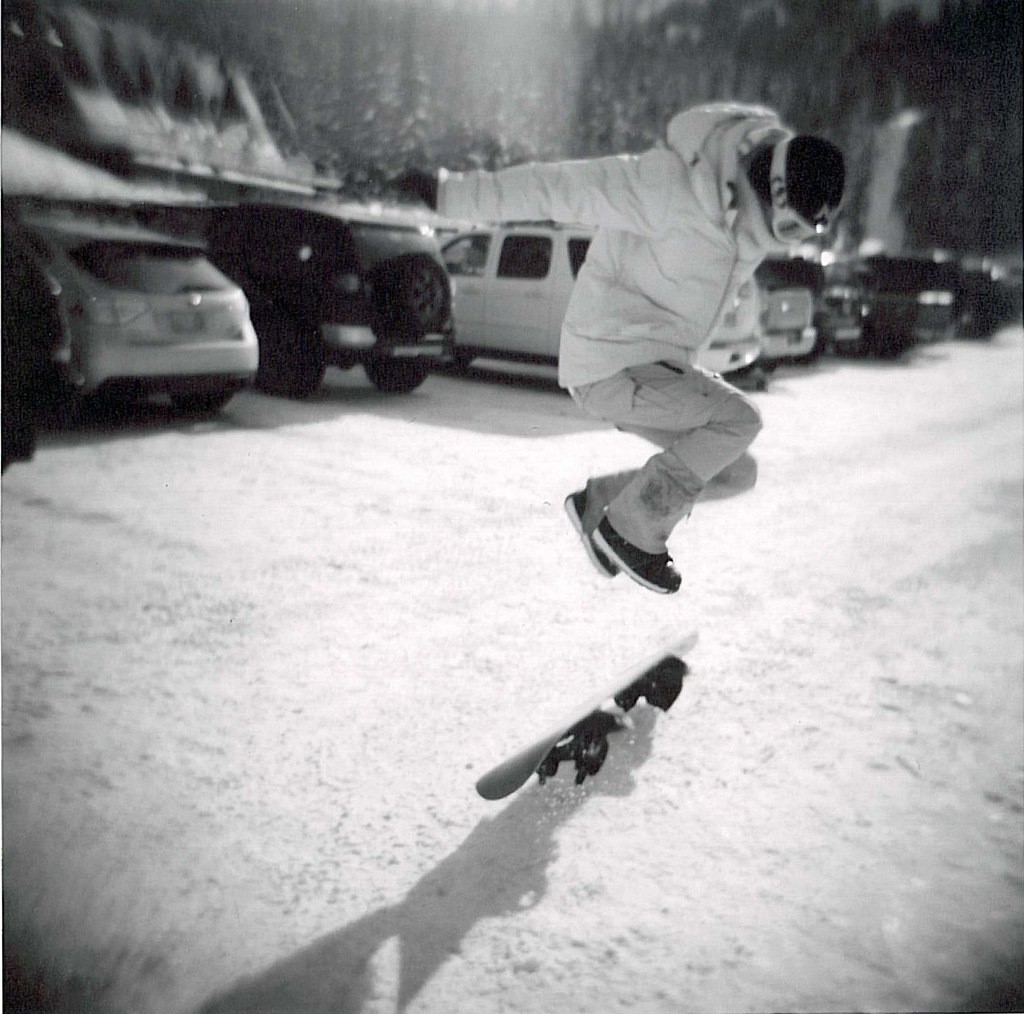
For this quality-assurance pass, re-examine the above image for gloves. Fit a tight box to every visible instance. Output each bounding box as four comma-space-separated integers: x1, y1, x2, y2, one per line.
384, 166, 438, 211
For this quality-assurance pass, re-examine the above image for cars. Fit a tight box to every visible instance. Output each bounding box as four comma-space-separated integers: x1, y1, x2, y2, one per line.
2, 229, 91, 483
756, 248, 1024, 369
12, 214, 263, 429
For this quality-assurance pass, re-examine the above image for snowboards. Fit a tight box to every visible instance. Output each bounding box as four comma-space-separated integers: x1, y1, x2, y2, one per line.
475, 629, 701, 801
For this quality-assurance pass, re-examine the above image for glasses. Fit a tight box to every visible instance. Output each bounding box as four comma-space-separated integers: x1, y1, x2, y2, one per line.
771, 136, 845, 247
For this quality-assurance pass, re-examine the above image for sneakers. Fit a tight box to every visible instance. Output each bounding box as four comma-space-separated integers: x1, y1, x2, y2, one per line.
590, 511, 682, 596
564, 488, 623, 583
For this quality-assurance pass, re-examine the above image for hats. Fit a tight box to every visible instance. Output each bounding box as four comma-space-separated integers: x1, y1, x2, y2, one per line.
745, 136, 845, 221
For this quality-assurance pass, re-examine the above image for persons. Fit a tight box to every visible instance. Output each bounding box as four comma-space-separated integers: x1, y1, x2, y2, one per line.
384, 100, 852, 598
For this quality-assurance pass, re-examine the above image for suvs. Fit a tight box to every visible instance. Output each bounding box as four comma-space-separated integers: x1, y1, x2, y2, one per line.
136, 202, 454, 404
436, 219, 762, 373
